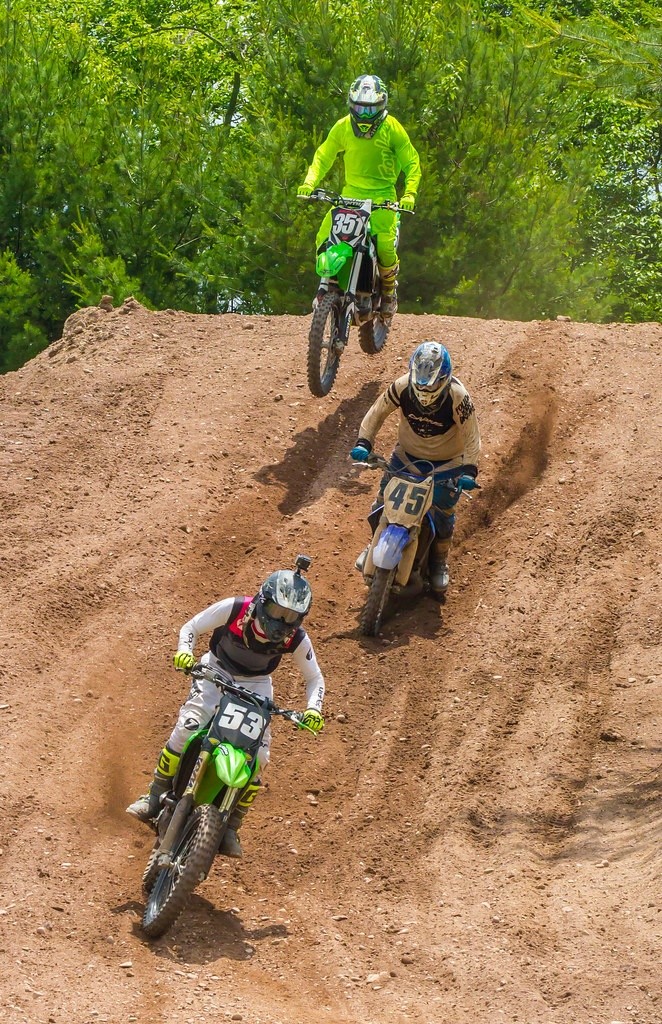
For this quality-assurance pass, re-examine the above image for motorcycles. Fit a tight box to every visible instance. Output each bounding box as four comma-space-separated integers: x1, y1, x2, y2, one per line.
348, 450, 483, 633
307, 187, 415, 396
139, 654, 321, 940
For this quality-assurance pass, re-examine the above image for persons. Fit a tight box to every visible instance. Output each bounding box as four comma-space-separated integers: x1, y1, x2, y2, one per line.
351, 342, 481, 593
126, 570, 326, 857
297, 73, 422, 318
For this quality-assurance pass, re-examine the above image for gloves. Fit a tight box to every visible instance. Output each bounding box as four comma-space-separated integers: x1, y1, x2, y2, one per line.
297, 184, 314, 196
173, 651, 195, 669
455, 475, 475, 496
350, 445, 370, 462
399, 193, 415, 211
298, 709, 325, 731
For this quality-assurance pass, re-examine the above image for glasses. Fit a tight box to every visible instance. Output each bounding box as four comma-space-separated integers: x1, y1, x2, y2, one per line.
263, 599, 301, 625
415, 378, 442, 392
349, 102, 385, 117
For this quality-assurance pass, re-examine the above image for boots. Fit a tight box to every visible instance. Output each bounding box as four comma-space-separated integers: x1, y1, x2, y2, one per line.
125, 741, 184, 823
219, 779, 262, 858
377, 254, 401, 319
354, 501, 383, 571
431, 536, 454, 592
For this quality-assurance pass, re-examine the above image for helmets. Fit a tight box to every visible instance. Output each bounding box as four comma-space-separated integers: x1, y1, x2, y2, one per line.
409, 341, 452, 408
348, 74, 389, 139
257, 569, 313, 643
